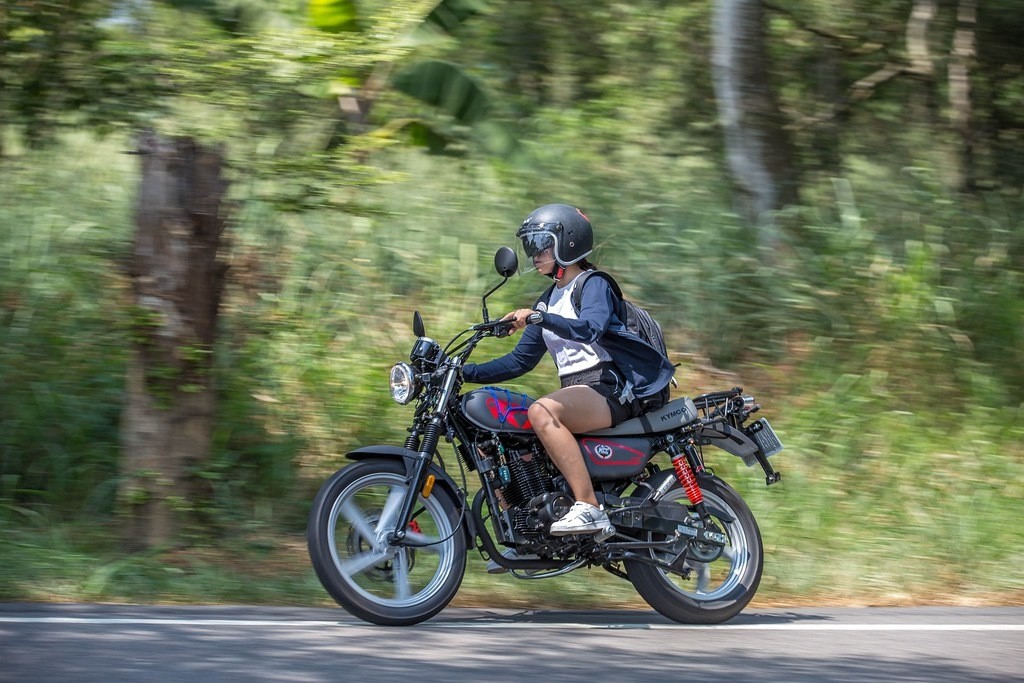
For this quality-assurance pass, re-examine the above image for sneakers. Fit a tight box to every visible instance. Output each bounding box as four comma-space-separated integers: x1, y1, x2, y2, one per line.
549, 501, 611, 536
487, 548, 540, 574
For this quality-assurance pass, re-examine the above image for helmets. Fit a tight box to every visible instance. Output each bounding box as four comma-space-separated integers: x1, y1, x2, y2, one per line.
515, 204, 593, 266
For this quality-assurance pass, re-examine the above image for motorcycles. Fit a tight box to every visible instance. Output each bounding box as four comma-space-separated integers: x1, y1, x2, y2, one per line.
306, 247, 784, 629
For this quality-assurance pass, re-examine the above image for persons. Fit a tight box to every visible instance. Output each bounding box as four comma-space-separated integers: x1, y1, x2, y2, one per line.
457, 203, 674, 573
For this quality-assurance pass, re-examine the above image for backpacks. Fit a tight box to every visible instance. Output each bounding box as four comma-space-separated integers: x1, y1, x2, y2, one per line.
540, 270, 681, 418
